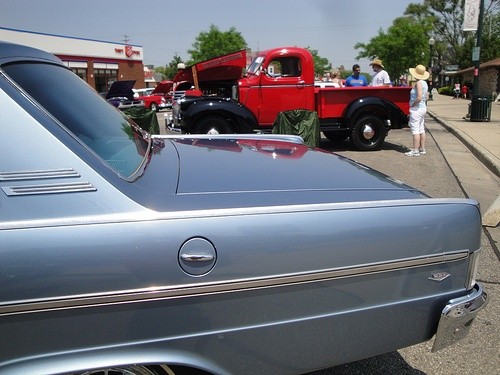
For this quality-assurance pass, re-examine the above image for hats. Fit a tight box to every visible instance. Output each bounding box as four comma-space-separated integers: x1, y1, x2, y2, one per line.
369, 59, 384, 68
409, 65, 429, 79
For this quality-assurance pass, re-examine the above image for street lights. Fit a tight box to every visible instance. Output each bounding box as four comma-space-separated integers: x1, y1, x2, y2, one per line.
427, 37, 436, 101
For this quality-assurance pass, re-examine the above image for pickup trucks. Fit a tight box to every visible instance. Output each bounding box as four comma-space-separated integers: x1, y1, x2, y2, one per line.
179, 44, 420, 153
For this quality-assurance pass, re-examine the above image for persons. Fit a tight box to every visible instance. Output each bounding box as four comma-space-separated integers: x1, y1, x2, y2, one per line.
404, 65, 430, 157
314, 59, 473, 99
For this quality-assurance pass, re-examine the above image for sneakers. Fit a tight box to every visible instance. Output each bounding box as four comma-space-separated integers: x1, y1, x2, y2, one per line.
410, 149, 426, 154
405, 150, 420, 156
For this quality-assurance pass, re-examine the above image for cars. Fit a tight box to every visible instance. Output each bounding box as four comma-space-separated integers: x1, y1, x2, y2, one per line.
0, 38, 488, 374
95, 72, 365, 114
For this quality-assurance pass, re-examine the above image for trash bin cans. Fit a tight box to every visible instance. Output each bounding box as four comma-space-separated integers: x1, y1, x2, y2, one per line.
469, 97, 489, 121
